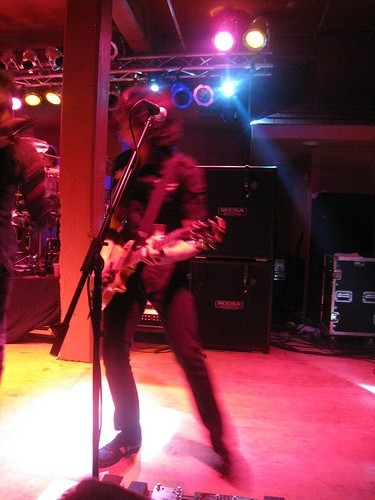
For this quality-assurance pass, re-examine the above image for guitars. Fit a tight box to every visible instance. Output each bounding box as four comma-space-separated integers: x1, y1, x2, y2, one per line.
102, 215, 228, 300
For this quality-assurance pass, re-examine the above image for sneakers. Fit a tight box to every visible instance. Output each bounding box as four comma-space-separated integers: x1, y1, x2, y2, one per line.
206, 435, 230, 475
97, 432, 142, 468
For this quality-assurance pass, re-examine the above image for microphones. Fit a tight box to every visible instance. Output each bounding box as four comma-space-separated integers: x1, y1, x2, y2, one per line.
142, 99, 167, 122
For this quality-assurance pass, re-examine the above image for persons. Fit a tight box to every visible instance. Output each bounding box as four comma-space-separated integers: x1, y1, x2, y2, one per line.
99, 86, 255, 487
1, 72, 60, 386
203, 113, 305, 340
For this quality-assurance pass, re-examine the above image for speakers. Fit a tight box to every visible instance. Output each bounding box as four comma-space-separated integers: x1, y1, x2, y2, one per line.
193, 165, 278, 261
187, 258, 273, 353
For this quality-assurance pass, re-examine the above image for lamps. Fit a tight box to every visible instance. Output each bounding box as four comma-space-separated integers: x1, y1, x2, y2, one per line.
168, 77, 216, 110
209, 12, 269, 53
0, 47, 64, 110
